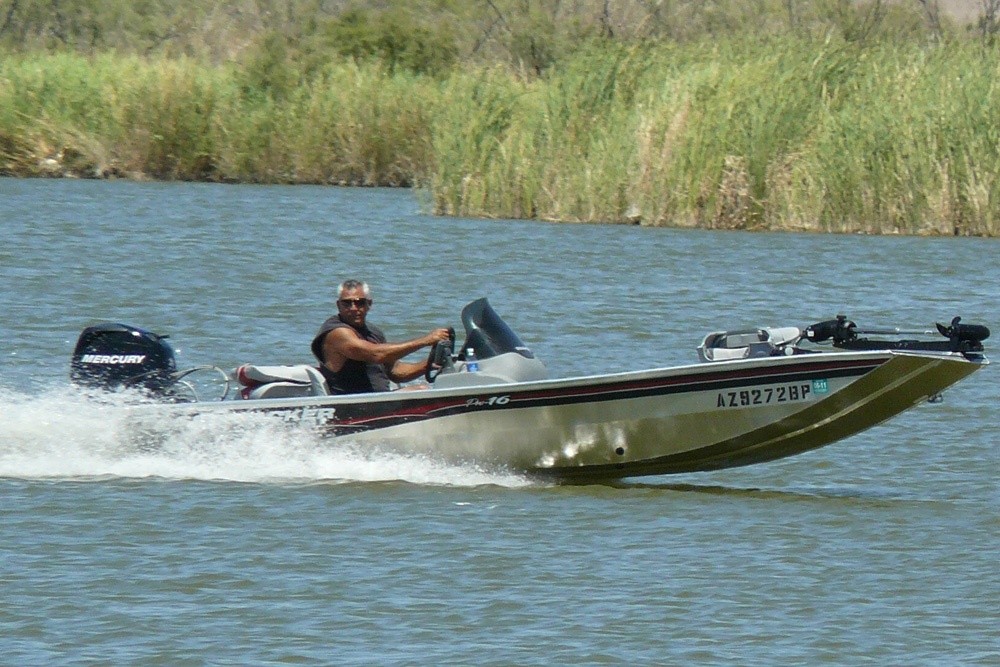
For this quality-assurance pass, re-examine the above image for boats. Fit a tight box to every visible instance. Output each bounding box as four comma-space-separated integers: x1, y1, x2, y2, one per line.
64, 296, 992, 482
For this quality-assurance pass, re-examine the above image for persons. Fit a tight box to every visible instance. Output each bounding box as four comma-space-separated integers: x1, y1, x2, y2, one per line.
310, 279, 448, 397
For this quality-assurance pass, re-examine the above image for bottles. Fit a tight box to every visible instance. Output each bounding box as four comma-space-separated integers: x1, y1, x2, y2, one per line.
465, 348, 480, 372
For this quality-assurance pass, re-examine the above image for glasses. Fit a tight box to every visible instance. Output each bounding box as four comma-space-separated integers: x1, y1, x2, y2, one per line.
339, 297, 369, 307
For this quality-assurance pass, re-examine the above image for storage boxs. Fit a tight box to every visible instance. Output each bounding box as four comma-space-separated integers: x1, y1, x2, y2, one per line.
69, 321, 177, 387
